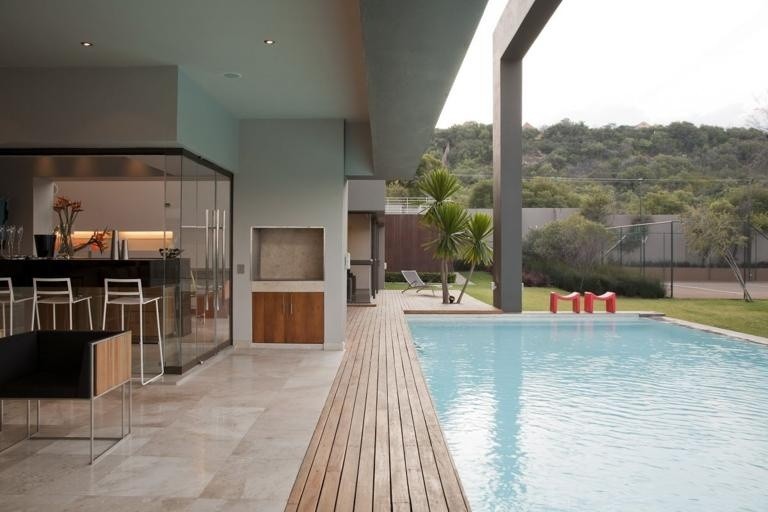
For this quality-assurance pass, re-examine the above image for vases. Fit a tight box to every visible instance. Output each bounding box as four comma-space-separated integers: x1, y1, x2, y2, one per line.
56, 224, 73, 259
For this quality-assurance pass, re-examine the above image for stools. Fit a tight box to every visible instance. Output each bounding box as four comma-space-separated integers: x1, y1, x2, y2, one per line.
584, 291, 617, 313
549, 291, 581, 313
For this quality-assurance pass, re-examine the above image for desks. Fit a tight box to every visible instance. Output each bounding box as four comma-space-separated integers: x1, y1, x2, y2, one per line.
0, 329, 132, 466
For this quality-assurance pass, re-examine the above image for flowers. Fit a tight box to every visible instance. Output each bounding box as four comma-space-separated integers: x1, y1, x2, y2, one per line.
53, 194, 112, 256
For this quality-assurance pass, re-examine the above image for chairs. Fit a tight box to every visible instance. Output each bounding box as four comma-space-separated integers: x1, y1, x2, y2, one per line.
29, 276, 95, 331
101, 278, 166, 385
400, 269, 453, 296
188, 269, 223, 327
0, 277, 41, 337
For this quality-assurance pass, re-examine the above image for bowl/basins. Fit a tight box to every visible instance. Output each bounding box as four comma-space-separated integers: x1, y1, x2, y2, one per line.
159, 248, 184, 258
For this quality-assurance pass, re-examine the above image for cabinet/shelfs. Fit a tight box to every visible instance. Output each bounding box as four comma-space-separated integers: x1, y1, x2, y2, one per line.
121, 285, 174, 343
78, 287, 122, 332
38, 286, 76, 328
250, 291, 325, 345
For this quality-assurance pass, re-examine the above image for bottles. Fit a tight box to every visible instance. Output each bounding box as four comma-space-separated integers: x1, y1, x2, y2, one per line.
120, 239, 129, 259
111, 229, 120, 259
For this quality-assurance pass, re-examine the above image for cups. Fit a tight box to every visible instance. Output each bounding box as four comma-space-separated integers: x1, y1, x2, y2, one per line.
0, 224, 24, 259
88, 247, 93, 258
